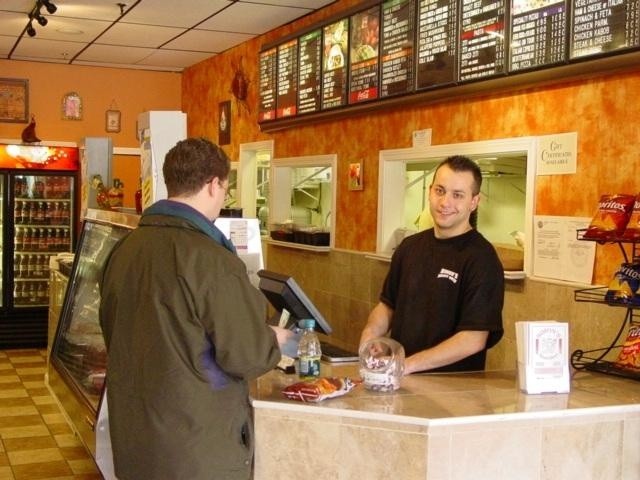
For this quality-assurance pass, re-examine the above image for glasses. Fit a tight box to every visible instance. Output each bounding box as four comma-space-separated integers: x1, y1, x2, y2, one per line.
205, 176, 233, 202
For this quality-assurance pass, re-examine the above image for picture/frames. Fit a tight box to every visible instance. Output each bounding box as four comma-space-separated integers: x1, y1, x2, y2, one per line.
347, 160, 363, 192
106, 109, 121, 132
62, 91, 83, 120
0, 77, 29, 123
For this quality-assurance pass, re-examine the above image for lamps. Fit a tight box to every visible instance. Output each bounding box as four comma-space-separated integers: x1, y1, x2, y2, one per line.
26, 0, 57, 37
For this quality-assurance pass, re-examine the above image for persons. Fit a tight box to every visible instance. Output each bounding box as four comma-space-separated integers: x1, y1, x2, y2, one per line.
218, 104, 228, 131
357, 157, 505, 377
100, 136, 294, 480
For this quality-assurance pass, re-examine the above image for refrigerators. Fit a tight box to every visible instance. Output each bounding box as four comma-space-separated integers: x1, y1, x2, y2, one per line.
0, 142, 81, 348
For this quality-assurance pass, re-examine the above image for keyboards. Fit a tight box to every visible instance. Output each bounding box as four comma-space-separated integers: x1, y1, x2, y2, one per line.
320, 340, 360, 364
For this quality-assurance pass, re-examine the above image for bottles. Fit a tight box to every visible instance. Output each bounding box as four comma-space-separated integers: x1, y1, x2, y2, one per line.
109, 178, 123, 209
14, 201, 68, 224
135, 174, 143, 215
17, 177, 69, 197
13, 283, 48, 304
14, 254, 49, 276
296, 319, 322, 381
14, 228, 69, 252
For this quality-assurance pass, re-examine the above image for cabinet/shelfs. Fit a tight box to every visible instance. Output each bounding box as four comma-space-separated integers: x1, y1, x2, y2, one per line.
570, 228, 640, 381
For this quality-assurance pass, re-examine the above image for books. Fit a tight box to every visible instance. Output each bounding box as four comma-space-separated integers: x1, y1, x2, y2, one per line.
514, 319, 570, 394
